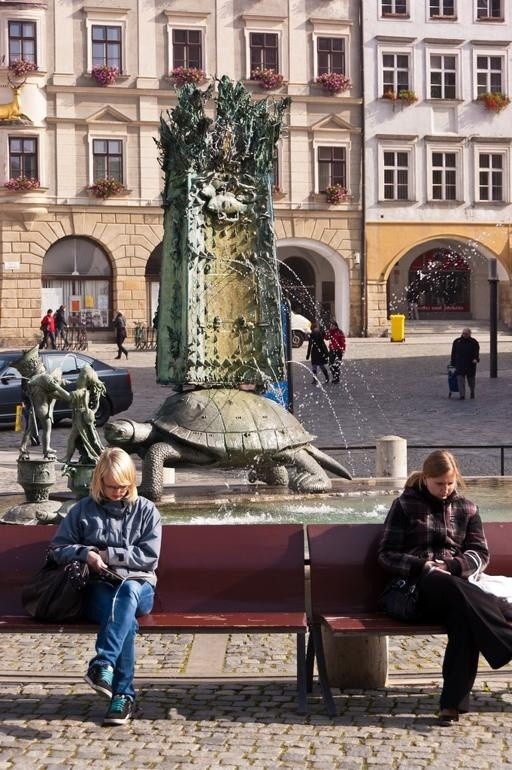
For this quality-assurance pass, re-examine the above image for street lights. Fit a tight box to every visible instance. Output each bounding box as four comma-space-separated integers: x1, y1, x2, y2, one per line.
486, 258, 500, 378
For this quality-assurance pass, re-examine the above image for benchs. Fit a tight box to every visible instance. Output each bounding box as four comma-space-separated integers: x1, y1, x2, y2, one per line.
0, 520, 309, 718
306, 521, 512, 723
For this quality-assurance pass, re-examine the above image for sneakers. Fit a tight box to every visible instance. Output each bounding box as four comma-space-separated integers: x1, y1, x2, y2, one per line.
104, 693, 135, 724
85, 664, 113, 699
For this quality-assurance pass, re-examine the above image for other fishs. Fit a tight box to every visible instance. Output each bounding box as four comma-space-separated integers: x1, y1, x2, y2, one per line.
206, 192, 254, 214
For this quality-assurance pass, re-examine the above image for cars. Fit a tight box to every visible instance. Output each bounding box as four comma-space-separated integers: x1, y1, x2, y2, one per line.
290, 312, 312, 347
1, 350, 134, 428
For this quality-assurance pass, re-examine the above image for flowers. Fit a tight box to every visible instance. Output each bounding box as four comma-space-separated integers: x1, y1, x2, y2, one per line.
323, 183, 353, 205
168, 66, 208, 90
5, 174, 43, 194
86, 176, 129, 204
8, 58, 37, 75
478, 89, 512, 112
314, 71, 353, 95
90, 65, 118, 88
271, 184, 285, 201
251, 67, 288, 91
384, 87, 421, 107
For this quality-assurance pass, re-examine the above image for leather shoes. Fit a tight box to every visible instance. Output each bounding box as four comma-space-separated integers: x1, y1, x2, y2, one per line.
439, 705, 458, 721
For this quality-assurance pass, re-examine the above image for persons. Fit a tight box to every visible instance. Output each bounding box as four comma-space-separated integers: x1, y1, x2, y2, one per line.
305, 323, 329, 385
52, 364, 104, 464
18, 373, 41, 446
449, 327, 479, 401
110, 309, 128, 360
407, 287, 419, 320
40, 309, 57, 350
324, 320, 346, 384
363, 450, 512, 726
10, 343, 58, 460
46, 444, 164, 727
53, 304, 69, 345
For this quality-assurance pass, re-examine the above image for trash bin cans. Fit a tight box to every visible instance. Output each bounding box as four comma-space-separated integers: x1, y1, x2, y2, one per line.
389, 312, 406, 342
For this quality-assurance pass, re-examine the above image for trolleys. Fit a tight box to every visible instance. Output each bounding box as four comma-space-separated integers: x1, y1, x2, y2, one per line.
447, 365, 458, 397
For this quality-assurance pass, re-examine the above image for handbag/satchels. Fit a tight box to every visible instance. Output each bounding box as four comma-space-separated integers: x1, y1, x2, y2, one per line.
468, 571, 512, 620
374, 587, 422, 623
22, 560, 90, 624
